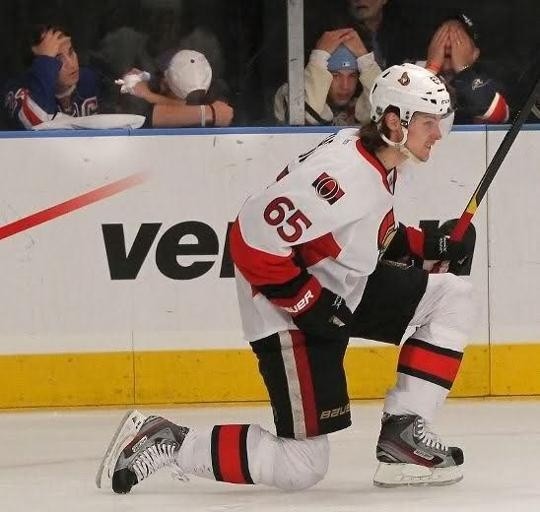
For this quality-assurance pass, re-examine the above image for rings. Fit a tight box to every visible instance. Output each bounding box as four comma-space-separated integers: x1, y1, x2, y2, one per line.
457, 42, 462, 45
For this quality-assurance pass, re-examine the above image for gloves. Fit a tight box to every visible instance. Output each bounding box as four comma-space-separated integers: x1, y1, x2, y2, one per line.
250, 263, 356, 349
398, 215, 479, 277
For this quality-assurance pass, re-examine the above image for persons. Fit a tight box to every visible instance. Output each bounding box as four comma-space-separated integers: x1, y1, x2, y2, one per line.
1, 21, 147, 132
115, 46, 235, 128
273, 27, 384, 126
402, 8, 510, 125
347, 0, 395, 70
96, 60, 478, 494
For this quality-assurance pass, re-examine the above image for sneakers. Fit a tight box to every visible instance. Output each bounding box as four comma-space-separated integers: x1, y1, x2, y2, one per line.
93, 406, 191, 496
372, 408, 466, 490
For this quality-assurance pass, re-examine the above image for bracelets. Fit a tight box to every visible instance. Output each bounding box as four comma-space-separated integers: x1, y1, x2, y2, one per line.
200, 104, 206, 128
208, 103, 217, 125
456, 65, 471, 71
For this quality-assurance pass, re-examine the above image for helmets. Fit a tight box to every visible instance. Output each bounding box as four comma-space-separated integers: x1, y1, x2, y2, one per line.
365, 61, 452, 131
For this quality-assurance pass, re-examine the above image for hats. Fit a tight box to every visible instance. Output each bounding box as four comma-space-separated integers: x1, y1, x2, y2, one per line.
325, 42, 361, 73
425, 9, 481, 49
162, 48, 214, 102
